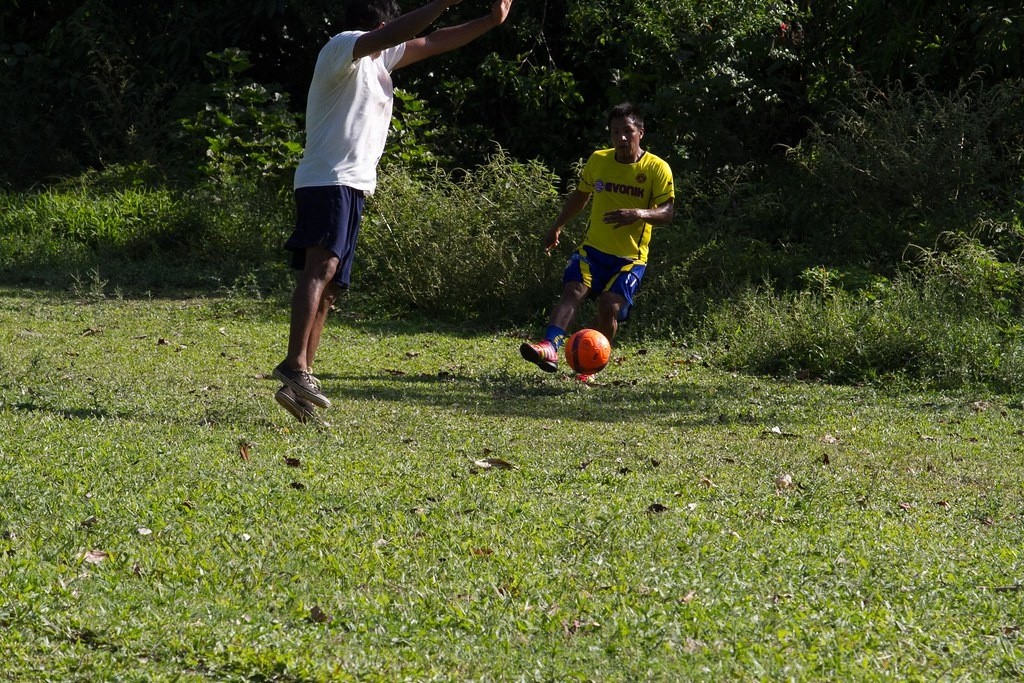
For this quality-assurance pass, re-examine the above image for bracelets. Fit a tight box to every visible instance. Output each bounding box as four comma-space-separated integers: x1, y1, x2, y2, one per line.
549, 225, 561, 234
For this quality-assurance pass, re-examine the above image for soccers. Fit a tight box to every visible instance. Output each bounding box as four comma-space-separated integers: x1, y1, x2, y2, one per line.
564, 327, 613, 375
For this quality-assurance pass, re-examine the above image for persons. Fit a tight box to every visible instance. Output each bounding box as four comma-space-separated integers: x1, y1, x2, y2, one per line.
271, 0, 514, 427
520, 102, 675, 385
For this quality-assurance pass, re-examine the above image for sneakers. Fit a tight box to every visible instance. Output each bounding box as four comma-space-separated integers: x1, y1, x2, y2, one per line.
519, 339, 557, 374
276, 382, 327, 432
273, 359, 329, 409
571, 370, 598, 385
